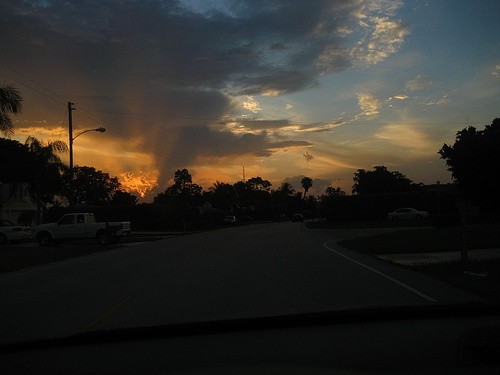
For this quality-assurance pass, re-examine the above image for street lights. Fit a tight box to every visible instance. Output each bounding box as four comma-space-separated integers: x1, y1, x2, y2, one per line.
67, 102, 106, 205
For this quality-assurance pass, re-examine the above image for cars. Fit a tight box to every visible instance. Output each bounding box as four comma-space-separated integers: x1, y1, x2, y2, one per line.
0, 219, 32, 245
293, 213, 305, 222
223, 215, 237, 223
387, 208, 431, 222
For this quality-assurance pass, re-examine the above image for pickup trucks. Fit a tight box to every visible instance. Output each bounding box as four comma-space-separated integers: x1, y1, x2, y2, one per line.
32, 212, 132, 249
